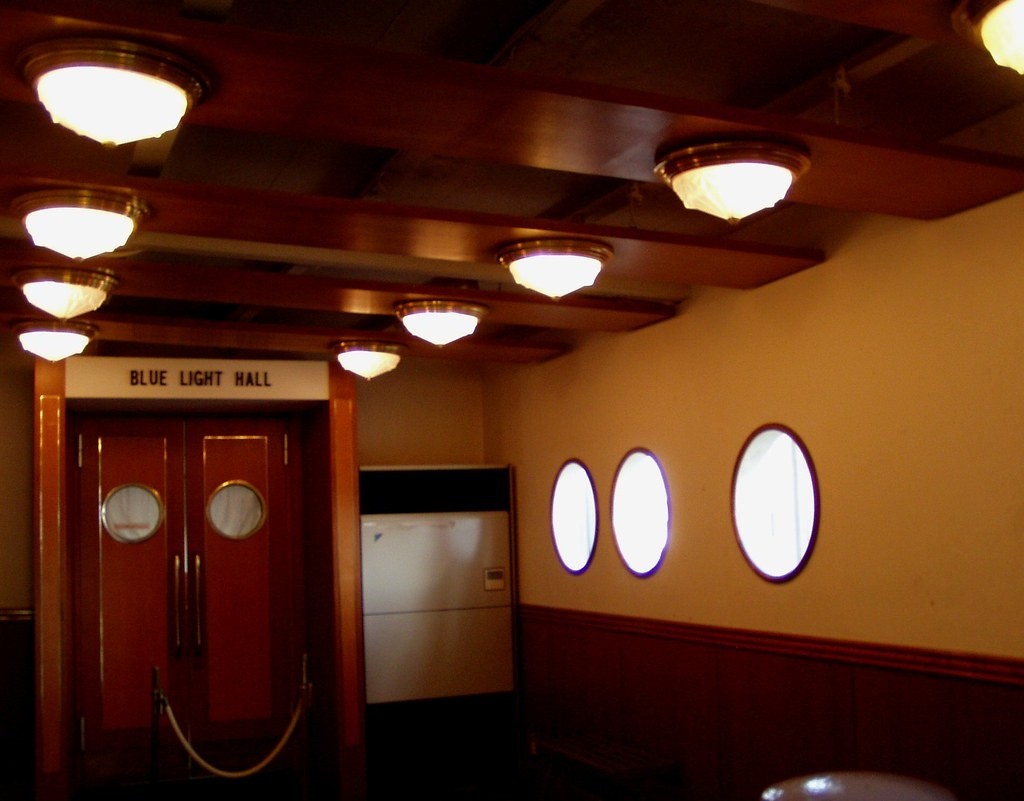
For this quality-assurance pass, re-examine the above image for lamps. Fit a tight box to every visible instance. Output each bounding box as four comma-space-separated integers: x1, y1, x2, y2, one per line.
11, 268, 119, 324
493, 238, 615, 302
649, 142, 811, 225
12, 189, 151, 265
951, 0, 1024, 76
11, 320, 100, 364
394, 301, 489, 349
330, 340, 410, 381
10, 33, 218, 151
